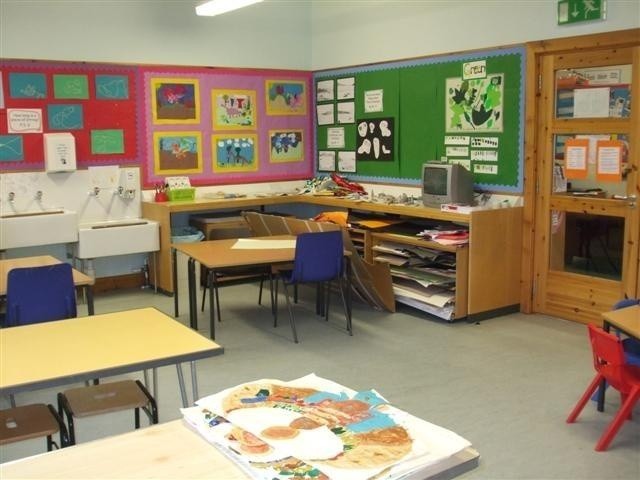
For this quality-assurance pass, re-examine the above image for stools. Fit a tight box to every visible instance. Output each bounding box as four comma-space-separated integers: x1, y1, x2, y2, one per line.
0, 403, 68, 452
55, 378, 159, 447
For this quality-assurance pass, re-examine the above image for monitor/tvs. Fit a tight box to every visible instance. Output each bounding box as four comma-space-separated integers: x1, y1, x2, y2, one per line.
421, 163, 472, 208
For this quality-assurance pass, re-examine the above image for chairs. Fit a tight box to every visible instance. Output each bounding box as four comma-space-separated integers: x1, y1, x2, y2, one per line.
199, 221, 278, 322
5, 263, 92, 413
272, 229, 352, 343
566, 322, 640, 453
593, 298, 640, 402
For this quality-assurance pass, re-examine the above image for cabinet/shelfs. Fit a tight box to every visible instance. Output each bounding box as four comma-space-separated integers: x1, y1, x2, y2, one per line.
140, 188, 527, 323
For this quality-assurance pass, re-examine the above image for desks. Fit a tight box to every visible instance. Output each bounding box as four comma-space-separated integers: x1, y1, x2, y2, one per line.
0, 375, 480, 479
0, 307, 225, 406
169, 232, 354, 342
598, 305, 640, 413
0, 254, 98, 314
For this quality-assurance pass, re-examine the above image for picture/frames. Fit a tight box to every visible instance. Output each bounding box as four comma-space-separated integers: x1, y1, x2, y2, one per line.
148, 70, 308, 175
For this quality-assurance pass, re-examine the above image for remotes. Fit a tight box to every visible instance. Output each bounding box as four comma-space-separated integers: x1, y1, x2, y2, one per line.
427, 160, 440, 164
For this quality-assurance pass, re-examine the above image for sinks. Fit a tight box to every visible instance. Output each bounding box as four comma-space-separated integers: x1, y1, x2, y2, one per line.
78, 217, 162, 260
0, 209, 79, 251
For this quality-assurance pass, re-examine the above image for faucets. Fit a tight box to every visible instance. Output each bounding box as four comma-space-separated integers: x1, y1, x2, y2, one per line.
34, 190, 43, 199
8, 192, 15, 201
118, 185, 124, 196
94, 186, 100, 196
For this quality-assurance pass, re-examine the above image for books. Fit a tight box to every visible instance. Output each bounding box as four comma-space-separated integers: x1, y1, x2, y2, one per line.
441, 204, 481, 213
312, 192, 334, 195
